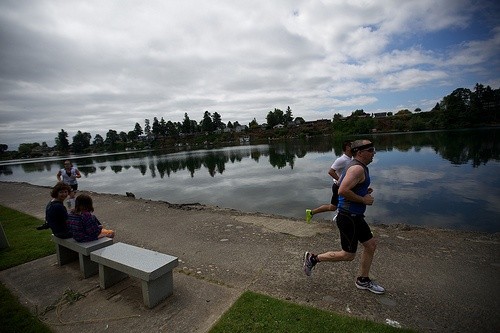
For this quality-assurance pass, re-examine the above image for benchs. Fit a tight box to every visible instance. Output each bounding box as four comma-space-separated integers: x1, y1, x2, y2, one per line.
90, 242, 179, 310
51, 234, 116, 279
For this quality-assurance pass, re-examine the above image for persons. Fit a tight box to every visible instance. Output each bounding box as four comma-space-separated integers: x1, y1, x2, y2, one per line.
304, 141, 385, 294
46, 182, 74, 238
67, 195, 114, 242
306, 140, 353, 224
57, 160, 81, 209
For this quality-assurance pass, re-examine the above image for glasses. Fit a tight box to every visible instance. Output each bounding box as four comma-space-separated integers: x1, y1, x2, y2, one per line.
364, 148, 375, 152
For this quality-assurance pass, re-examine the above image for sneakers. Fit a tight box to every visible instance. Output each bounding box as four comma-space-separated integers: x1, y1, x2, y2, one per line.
303, 251, 313, 277
305, 209, 313, 224
355, 277, 386, 294
